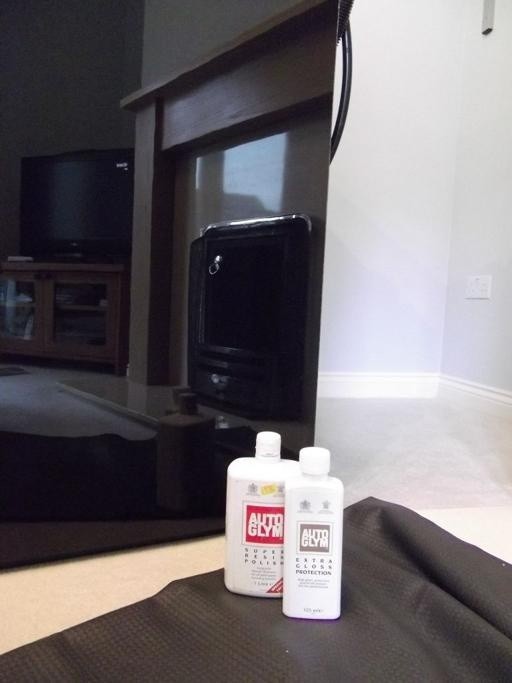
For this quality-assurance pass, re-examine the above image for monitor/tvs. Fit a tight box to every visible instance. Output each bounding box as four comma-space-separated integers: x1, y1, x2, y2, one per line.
19, 148, 134, 262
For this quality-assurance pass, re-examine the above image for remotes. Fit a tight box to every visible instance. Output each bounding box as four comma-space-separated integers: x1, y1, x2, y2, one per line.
8, 256, 34, 261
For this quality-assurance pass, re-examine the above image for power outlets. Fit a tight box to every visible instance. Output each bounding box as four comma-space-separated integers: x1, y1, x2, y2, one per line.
465, 271, 491, 297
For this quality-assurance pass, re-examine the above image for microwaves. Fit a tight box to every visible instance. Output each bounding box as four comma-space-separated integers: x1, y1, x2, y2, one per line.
186, 214, 314, 422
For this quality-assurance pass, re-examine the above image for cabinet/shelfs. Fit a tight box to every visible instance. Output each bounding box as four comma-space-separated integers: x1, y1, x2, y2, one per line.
0, 263, 129, 377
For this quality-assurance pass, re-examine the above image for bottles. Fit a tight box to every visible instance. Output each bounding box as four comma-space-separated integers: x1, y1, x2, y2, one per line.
222, 428, 291, 600
156, 385, 220, 518
279, 446, 347, 620
